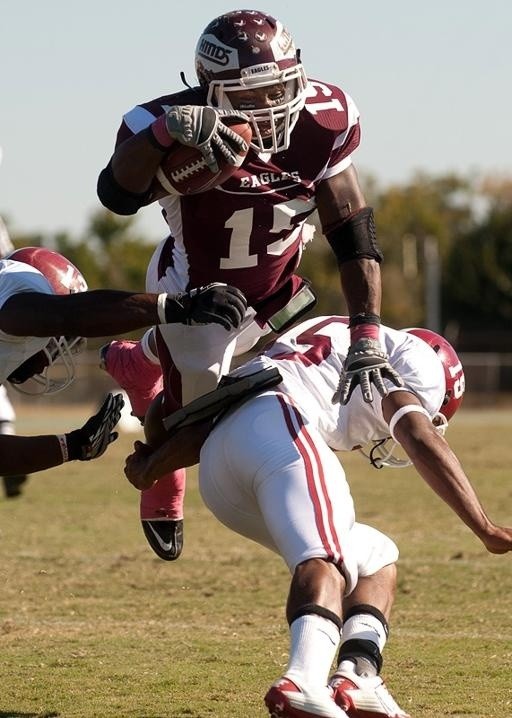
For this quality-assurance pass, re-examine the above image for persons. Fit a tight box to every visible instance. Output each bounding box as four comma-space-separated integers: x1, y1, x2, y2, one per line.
95, 7, 404, 560
0, 244, 251, 481
122, 312, 512, 716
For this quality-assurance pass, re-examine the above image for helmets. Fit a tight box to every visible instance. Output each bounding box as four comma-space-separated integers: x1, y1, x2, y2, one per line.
394, 327, 467, 437
3, 247, 89, 349
194, 7, 308, 119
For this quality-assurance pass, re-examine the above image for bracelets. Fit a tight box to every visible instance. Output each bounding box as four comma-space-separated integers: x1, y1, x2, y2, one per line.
54, 433, 69, 463
156, 291, 169, 324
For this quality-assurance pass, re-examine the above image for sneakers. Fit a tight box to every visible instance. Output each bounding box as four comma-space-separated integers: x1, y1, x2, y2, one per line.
98, 339, 165, 426
139, 468, 186, 562
259, 667, 408, 717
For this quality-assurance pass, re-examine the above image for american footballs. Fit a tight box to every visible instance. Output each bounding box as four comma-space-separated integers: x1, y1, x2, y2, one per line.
156, 116, 253, 195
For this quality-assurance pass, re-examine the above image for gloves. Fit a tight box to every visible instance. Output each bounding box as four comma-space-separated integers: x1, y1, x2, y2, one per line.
122, 439, 183, 491
164, 104, 254, 177
330, 332, 405, 407
164, 281, 249, 332
65, 391, 128, 463
